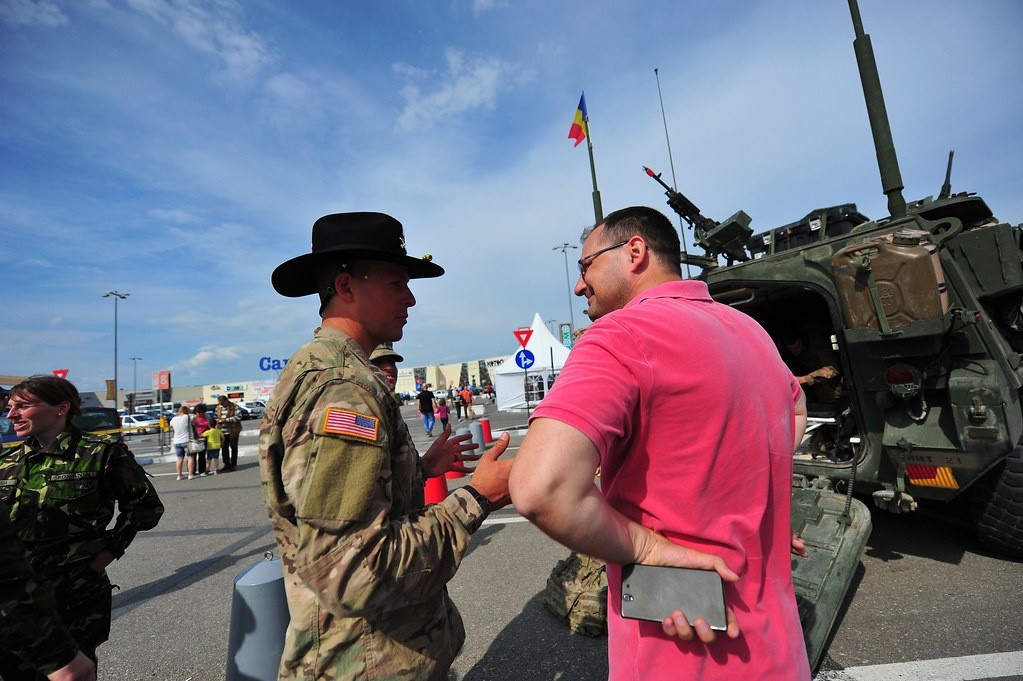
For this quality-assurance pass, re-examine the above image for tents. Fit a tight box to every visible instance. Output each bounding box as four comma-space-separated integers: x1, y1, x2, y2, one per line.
493, 312, 571, 411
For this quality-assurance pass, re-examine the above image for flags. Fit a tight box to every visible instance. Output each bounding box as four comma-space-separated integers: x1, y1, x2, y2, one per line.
567, 94, 587, 148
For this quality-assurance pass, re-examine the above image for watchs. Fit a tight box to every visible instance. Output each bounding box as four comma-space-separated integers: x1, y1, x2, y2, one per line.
462, 485, 492, 515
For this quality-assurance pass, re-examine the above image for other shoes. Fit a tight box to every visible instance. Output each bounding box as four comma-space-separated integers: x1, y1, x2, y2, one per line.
426, 429, 432, 436
201, 472, 206, 477
187, 473, 199, 479
195, 471, 200, 474
177, 475, 186, 481
220, 463, 236, 472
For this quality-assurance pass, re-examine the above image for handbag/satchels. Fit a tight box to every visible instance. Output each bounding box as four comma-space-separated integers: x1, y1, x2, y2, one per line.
189, 440, 205, 453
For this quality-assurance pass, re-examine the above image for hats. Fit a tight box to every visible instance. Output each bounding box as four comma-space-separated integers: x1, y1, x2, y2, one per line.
272, 212, 445, 297
367, 339, 403, 362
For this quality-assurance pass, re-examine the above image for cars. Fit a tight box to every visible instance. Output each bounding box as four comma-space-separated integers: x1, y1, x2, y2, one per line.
400, 389, 449, 402
118, 399, 267, 437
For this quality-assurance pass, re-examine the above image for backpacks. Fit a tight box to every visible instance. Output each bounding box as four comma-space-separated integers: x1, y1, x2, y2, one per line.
542, 548, 608, 637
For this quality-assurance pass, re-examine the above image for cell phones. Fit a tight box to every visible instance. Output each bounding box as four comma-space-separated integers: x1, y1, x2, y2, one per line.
621, 563, 727, 631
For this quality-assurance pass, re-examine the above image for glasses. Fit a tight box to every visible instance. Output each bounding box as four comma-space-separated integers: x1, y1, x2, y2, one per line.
578, 242, 648, 278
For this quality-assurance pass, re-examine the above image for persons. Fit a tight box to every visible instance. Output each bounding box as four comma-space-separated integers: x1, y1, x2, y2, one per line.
446, 381, 474, 422
507, 207, 814, 681
259, 211, 514, 680
487, 383, 492, 396
169, 395, 242, 480
435, 398, 450, 432
416, 383, 438, 437
0, 373, 164, 681
367, 341, 404, 392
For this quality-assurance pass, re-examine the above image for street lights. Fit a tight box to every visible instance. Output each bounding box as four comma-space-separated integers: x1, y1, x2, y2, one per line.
102, 291, 128, 408
552, 242, 578, 330
